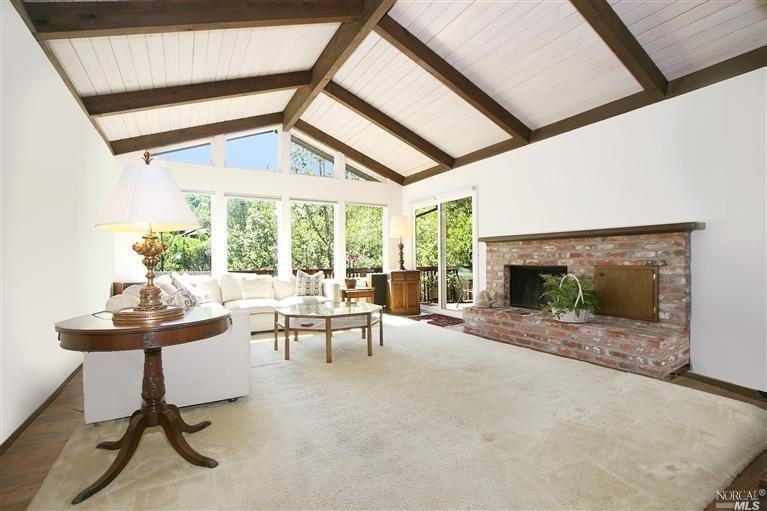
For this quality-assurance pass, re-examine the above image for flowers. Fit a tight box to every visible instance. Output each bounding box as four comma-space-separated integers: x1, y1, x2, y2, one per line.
346, 253, 359, 278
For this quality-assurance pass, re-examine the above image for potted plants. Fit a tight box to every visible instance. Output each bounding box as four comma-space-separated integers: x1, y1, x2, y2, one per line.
421, 272, 456, 298
537, 271, 602, 322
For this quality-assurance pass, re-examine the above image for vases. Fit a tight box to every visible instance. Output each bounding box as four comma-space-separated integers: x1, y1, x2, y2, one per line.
344, 278, 357, 288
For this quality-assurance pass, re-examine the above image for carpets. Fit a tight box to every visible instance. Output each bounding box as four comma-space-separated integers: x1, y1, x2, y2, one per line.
26, 314, 767, 510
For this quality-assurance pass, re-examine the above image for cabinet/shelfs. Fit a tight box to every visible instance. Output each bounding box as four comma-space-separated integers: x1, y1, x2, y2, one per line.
385, 269, 420, 314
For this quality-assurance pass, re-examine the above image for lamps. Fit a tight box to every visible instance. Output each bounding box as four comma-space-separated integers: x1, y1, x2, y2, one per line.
388, 216, 410, 271
93, 151, 202, 326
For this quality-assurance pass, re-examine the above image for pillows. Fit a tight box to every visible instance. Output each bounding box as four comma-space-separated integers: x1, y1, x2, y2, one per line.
163, 269, 325, 313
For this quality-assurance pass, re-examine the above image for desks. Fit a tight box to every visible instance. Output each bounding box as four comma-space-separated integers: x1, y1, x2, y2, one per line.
55, 307, 232, 505
340, 287, 375, 303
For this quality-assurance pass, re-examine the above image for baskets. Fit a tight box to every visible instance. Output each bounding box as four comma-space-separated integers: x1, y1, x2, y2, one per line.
551, 274, 591, 323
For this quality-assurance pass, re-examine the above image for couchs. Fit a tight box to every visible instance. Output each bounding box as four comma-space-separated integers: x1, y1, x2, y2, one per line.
82, 274, 339, 426
